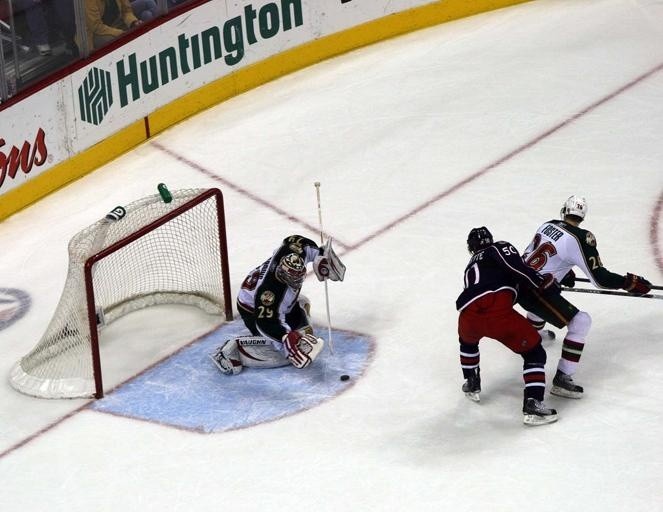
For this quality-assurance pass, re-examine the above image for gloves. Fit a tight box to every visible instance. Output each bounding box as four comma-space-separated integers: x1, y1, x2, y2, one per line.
559, 269, 576, 288
312, 236, 346, 283
621, 271, 651, 296
538, 272, 561, 297
281, 330, 324, 370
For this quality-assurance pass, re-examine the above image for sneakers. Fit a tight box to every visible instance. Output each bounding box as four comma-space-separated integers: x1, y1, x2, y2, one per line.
215, 339, 243, 376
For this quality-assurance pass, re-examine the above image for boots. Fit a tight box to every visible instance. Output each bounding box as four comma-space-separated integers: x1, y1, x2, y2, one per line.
462, 367, 481, 393
552, 368, 584, 393
521, 389, 557, 417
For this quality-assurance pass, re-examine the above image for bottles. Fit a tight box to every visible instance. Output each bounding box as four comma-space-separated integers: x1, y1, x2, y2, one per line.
156, 183, 172, 203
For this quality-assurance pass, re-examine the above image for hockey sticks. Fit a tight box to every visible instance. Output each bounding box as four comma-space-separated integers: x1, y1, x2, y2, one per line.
315, 181, 344, 356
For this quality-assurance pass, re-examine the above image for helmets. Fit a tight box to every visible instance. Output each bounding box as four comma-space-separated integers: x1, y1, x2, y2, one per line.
558, 194, 587, 223
274, 252, 306, 289
466, 225, 493, 256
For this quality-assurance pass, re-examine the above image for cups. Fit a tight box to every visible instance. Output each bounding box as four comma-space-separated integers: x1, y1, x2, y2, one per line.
104, 206, 126, 223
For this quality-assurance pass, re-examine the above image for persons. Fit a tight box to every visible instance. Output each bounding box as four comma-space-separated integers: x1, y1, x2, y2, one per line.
459, 223, 564, 423
211, 233, 343, 378
13, 0, 175, 59
515, 196, 651, 395
